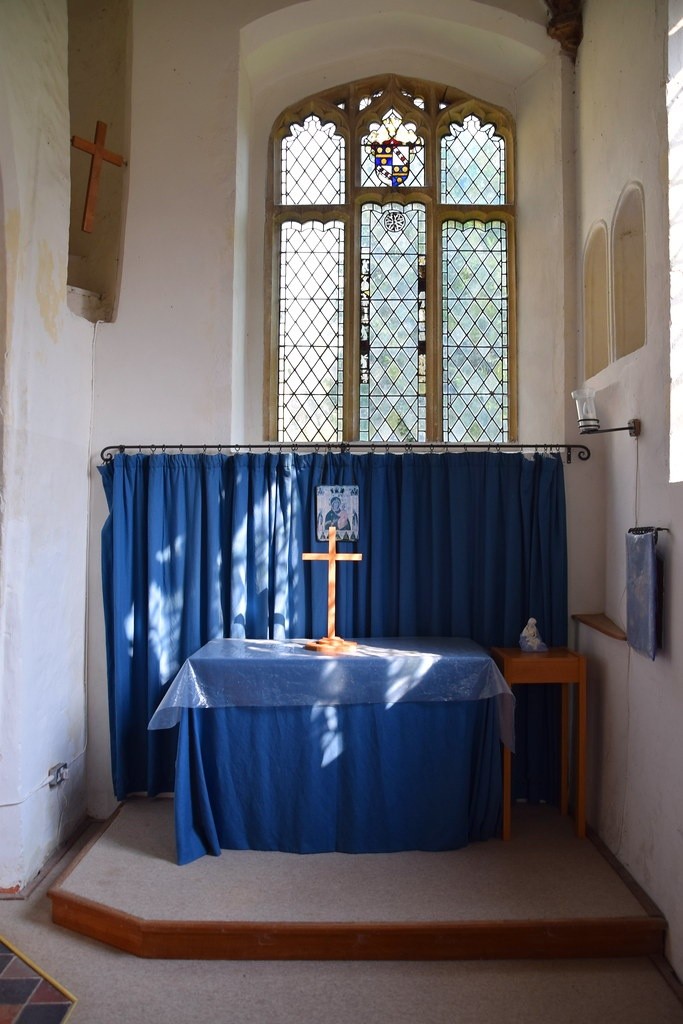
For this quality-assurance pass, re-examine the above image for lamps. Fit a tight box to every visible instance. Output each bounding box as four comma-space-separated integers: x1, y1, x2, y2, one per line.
570, 389, 640, 436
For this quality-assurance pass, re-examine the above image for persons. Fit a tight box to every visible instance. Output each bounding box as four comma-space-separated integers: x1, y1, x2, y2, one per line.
519, 618, 547, 650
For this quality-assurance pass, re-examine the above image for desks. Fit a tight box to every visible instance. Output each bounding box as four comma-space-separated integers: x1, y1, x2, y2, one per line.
488, 645, 591, 845
146, 638, 517, 870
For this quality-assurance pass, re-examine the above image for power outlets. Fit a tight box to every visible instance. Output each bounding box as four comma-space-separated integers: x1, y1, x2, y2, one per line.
50, 762, 67, 786
